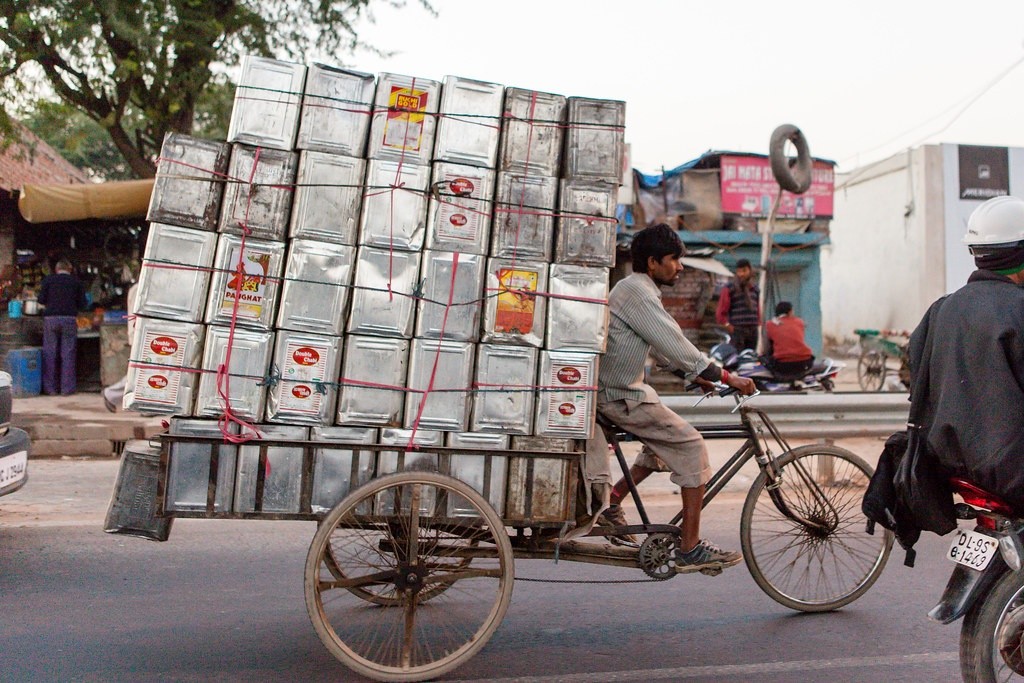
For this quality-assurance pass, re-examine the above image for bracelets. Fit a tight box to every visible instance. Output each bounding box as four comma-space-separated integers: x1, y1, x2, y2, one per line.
722, 369, 729, 385
725, 323, 729, 326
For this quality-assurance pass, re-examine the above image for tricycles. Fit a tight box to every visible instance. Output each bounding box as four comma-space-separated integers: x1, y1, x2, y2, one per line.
156, 378, 894, 683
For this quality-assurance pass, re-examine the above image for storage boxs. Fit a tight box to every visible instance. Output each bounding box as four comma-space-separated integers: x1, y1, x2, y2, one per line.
105, 58, 628, 543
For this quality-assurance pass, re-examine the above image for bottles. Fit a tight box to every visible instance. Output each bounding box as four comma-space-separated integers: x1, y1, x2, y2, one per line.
8, 300, 22, 319
24, 299, 39, 316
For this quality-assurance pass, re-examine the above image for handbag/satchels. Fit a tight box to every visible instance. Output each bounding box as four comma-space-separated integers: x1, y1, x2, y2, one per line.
861, 430, 957, 549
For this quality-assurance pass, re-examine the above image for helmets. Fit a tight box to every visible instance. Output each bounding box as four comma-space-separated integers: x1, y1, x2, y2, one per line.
962, 196, 1024, 246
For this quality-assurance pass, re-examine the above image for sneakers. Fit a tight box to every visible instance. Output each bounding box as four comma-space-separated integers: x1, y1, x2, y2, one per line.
675, 539, 741, 573
596, 504, 641, 547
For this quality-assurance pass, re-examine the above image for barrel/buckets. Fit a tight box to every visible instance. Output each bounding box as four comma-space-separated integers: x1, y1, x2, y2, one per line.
0, 348, 41, 395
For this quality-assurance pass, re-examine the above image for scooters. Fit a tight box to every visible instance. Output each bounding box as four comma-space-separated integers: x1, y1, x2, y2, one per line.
926, 475, 1024, 683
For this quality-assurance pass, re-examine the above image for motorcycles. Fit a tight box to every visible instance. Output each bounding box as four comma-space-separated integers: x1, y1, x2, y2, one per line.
708, 330, 847, 394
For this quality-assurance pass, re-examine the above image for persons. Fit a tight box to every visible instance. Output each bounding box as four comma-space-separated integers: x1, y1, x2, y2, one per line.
596, 223, 757, 575
717, 258, 816, 391
38, 260, 88, 396
908, 194, 1024, 520
